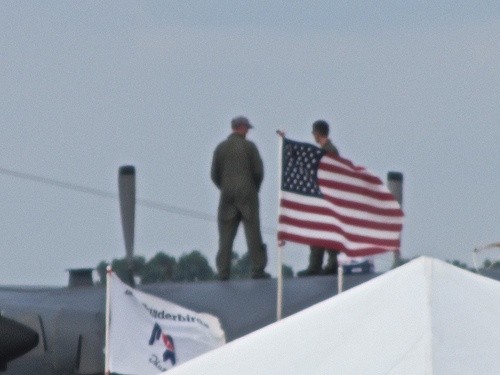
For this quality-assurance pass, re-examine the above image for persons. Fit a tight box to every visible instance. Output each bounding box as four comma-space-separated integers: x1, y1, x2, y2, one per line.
296, 121, 340, 277
211, 117, 270, 279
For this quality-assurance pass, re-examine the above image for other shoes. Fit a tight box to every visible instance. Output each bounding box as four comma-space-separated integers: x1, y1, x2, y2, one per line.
253, 270, 271, 280
219, 272, 229, 280
322, 267, 338, 275
297, 269, 321, 278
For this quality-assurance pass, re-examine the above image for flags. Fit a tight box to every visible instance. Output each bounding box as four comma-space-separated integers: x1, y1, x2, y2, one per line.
106, 271, 225, 374
277, 138, 404, 257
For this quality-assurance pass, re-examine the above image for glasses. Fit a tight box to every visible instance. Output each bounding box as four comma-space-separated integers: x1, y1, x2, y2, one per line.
312, 129, 319, 134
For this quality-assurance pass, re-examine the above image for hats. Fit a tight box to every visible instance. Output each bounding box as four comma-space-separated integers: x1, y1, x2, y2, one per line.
231, 117, 254, 129
